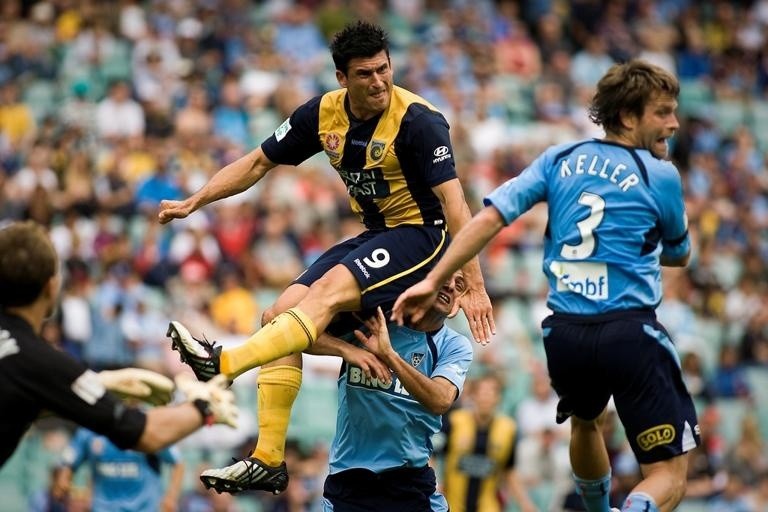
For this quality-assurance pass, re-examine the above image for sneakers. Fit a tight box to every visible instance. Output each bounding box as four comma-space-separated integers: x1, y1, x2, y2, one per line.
166, 321, 234, 391
199, 450, 290, 496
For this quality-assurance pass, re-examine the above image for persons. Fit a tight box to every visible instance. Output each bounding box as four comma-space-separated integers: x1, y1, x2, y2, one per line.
1, 1, 768, 511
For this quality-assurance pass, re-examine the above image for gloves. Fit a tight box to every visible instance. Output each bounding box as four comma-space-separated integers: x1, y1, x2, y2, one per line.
174, 370, 241, 430
98, 367, 174, 405
